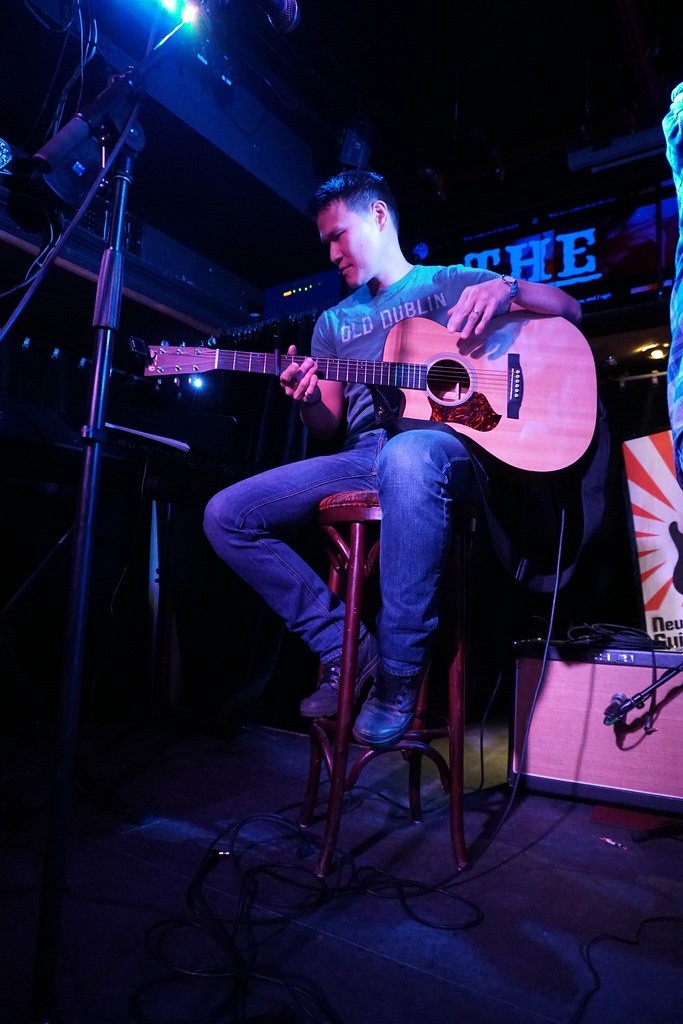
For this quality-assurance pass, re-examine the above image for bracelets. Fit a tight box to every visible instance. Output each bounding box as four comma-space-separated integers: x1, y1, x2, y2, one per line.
299, 397, 321, 408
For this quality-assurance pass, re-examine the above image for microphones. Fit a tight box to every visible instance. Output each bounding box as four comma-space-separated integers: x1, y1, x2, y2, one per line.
604, 693, 627, 723
250, 0, 301, 33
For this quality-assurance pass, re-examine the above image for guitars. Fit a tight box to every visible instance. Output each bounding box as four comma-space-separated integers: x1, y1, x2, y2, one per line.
142, 310, 597, 488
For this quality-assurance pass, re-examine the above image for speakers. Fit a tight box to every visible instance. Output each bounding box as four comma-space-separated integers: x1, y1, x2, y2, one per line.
505, 642, 683, 815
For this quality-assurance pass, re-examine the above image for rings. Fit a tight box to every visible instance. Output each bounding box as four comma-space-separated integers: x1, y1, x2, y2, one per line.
305, 392, 311, 399
473, 310, 481, 316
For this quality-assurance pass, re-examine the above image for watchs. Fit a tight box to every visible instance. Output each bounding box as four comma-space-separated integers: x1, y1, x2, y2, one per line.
501, 274, 518, 301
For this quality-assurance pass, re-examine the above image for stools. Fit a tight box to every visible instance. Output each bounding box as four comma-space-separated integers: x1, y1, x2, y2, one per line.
300, 486, 474, 880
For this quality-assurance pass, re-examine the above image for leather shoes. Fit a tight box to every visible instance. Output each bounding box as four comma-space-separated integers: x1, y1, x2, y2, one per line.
351, 657, 427, 748
300, 632, 378, 717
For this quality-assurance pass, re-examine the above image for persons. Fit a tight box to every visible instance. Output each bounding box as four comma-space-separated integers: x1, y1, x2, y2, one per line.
203, 169, 582, 748
